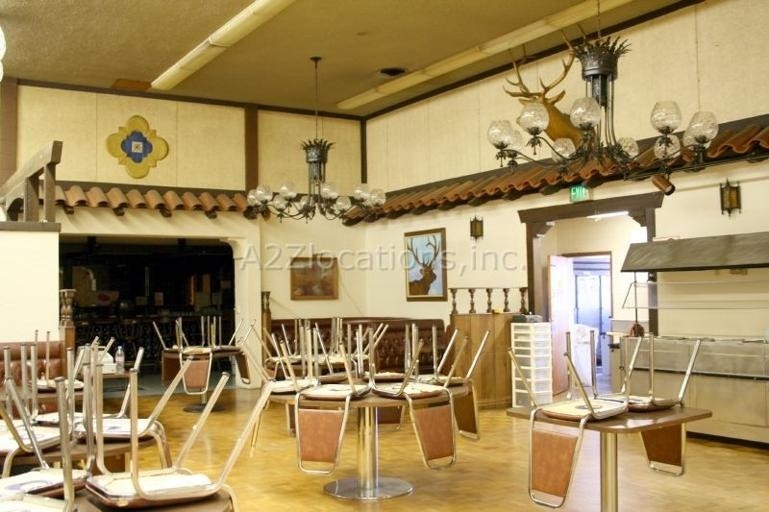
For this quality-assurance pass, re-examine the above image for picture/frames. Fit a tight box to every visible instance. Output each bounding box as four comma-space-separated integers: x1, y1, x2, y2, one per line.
403, 226, 450, 302
290, 255, 340, 302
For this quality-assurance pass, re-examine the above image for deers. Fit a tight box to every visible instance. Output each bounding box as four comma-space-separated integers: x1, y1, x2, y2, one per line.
502, 24, 596, 154
407, 235, 440, 295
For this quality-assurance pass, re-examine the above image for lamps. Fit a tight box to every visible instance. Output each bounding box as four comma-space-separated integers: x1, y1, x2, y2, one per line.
469, 216, 484, 241
245, 56, 389, 226
483, 0, 720, 176
718, 178, 742, 218
